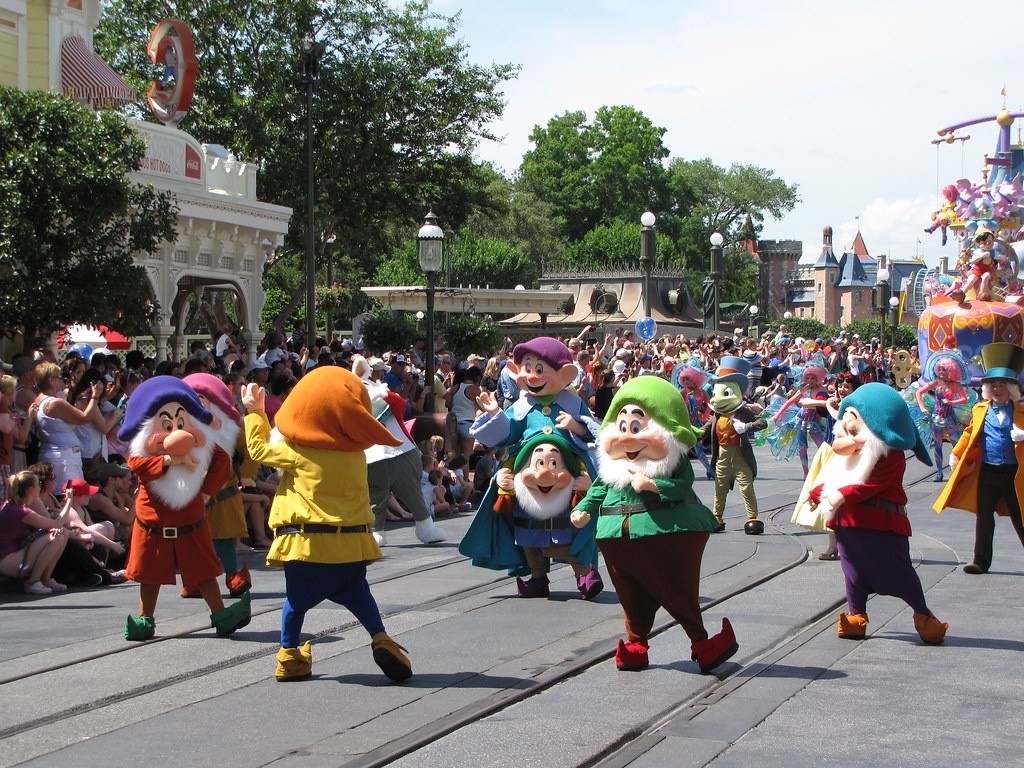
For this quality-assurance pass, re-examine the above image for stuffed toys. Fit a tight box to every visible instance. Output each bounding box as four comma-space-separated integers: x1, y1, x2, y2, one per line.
695, 356, 768, 536
457, 336, 739, 674
116, 354, 449, 684
803, 382, 949, 644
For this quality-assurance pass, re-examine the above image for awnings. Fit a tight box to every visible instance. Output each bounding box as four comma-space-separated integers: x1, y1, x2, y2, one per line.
61, 35, 136, 107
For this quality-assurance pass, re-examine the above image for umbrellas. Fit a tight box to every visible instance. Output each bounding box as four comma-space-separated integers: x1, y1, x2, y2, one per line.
58, 321, 131, 350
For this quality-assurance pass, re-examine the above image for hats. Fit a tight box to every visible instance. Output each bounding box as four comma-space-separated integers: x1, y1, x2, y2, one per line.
62, 478, 100, 495
813, 329, 881, 356
96, 461, 130, 481
13, 356, 36, 375
369, 353, 485, 374
733, 327, 744, 335
980, 342, 1024, 385
249, 359, 273, 372
568, 338, 583, 348
616, 348, 630, 359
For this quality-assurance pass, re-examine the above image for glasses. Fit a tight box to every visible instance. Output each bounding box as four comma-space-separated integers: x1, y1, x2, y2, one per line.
34, 481, 43, 489
52, 374, 62, 379
112, 473, 123, 479
46, 474, 57, 483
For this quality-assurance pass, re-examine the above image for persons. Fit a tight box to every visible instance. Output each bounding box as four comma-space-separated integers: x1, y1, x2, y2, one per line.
0, 318, 922, 595
915, 358, 969, 482
950, 225, 998, 301
798, 370, 863, 561
948, 367, 1024, 574
770, 366, 837, 482
924, 179, 984, 247
670, 356, 717, 481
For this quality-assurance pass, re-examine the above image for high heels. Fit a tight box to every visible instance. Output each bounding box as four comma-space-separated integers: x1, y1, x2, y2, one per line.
819, 546, 839, 561
933, 474, 944, 482
706, 472, 715, 480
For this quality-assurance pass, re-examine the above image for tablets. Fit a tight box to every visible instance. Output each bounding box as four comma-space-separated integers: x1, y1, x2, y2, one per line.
587, 339, 597, 349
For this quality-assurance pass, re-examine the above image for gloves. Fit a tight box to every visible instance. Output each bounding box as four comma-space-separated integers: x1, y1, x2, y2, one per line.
949, 455, 960, 472
1010, 423, 1024, 442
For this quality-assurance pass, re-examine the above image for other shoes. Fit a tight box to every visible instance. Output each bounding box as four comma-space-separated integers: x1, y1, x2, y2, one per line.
72, 573, 102, 587
453, 502, 472, 513
961, 563, 988, 577
111, 570, 129, 583
24, 577, 68, 595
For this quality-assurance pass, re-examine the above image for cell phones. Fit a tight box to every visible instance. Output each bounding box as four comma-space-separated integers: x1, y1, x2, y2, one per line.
198, 350, 209, 359
64, 479, 72, 492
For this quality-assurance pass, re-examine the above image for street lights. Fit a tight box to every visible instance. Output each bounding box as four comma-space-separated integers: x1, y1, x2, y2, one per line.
638, 212, 656, 318
888, 297, 899, 345
709, 232, 724, 329
417, 208, 446, 414
876, 269, 890, 352
